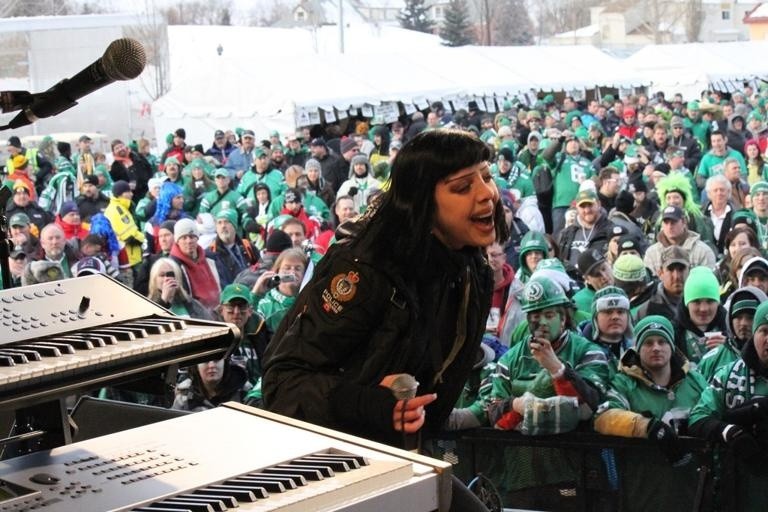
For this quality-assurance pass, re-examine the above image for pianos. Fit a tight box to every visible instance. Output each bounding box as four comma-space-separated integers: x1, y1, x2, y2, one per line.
0, 400, 452, 512
1, 271, 241, 411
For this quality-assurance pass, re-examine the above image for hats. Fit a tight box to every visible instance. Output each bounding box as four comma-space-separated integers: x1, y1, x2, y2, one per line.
305, 138, 377, 177
499, 189, 647, 340
7, 135, 130, 277
661, 181, 768, 331
687, 90, 768, 121
652, 163, 671, 177
588, 93, 684, 132
480, 95, 581, 161
220, 283, 252, 305
148, 128, 306, 253
635, 314, 676, 355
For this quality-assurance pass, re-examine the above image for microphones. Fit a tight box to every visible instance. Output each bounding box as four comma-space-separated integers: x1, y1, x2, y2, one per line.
390, 375, 420, 455
9, 37, 146, 130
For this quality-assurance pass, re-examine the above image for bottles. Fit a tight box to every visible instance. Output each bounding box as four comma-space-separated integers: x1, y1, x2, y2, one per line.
492, 410, 522, 431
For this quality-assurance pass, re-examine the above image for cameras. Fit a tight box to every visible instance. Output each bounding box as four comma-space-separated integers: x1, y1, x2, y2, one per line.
270, 274, 296, 286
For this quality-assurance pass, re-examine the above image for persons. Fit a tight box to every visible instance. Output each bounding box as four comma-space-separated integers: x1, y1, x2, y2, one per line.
1, 85, 766, 511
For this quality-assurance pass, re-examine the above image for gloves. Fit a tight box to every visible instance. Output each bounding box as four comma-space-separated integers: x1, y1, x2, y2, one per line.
647, 418, 677, 448
726, 426, 760, 458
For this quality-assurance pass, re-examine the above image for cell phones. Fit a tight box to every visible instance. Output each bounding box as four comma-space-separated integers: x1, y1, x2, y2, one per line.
166, 271, 174, 277
534, 330, 543, 345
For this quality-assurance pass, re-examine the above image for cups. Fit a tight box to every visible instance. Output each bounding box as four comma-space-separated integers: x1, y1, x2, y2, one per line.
484, 397, 509, 426
704, 332, 722, 350
231, 355, 247, 367
